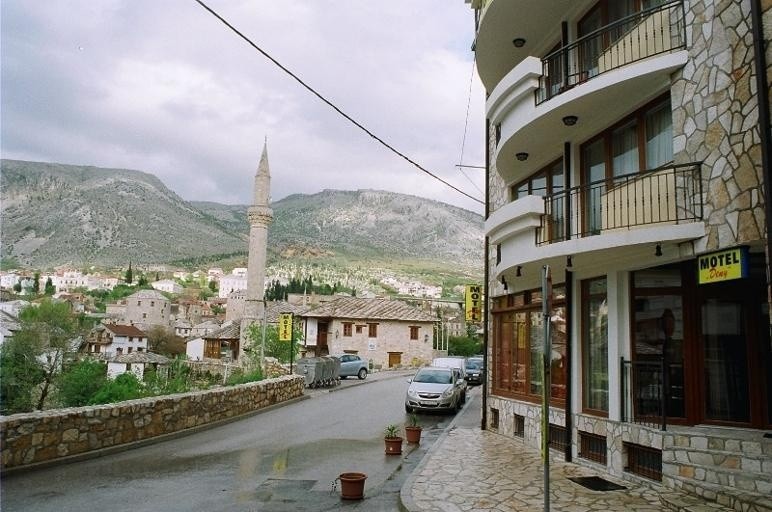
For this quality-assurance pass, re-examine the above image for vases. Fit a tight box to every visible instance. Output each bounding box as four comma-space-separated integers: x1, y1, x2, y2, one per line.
339, 472, 367, 501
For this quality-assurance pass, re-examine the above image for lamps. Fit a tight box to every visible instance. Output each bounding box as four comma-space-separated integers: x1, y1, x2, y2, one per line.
501, 275, 508, 295
653, 244, 665, 265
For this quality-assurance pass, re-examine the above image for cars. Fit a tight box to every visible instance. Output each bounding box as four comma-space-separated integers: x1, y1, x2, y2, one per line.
405, 356, 483, 415
326, 354, 370, 379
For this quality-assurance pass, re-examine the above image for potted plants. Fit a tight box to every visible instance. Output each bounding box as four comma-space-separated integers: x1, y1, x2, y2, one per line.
404, 412, 423, 443
384, 424, 403, 456
